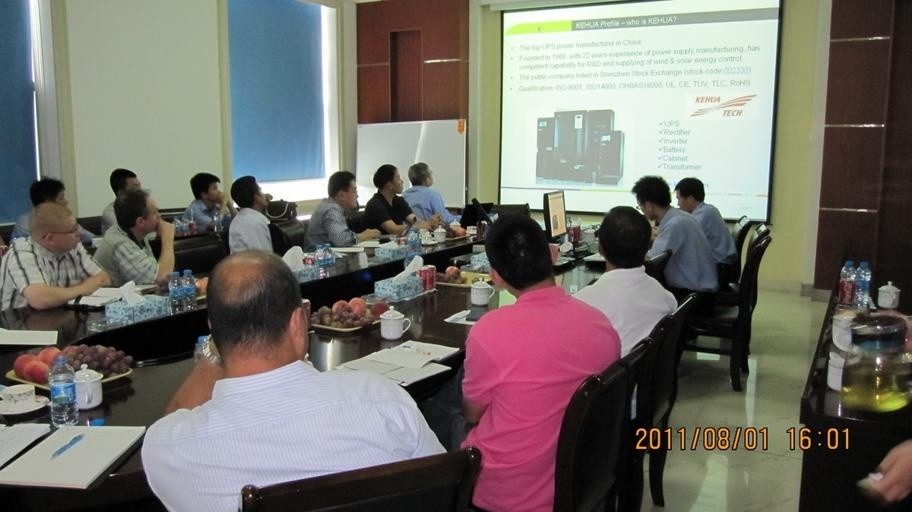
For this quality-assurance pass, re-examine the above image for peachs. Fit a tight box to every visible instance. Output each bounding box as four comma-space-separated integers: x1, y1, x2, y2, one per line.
334, 298, 389, 319
454, 227, 465, 237
15, 347, 61, 382
445, 266, 458, 272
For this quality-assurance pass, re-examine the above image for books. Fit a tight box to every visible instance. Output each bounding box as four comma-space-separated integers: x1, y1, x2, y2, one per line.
0, 420, 147, 496
66, 285, 121, 312
340, 336, 462, 388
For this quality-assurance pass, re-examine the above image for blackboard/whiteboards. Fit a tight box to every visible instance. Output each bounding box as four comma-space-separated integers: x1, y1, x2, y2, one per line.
355, 118, 467, 210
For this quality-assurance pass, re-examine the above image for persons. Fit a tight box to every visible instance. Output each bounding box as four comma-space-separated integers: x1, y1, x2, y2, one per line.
362, 163, 444, 236
228, 176, 276, 253
90, 187, 177, 287
11, 177, 95, 243
141, 250, 449, 511
401, 162, 466, 238
304, 170, 381, 251
99, 169, 160, 241
869, 436, 912, 503
0, 201, 112, 310
630, 174, 719, 321
570, 205, 681, 424
672, 178, 741, 287
182, 172, 237, 232
452, 214, 620, 512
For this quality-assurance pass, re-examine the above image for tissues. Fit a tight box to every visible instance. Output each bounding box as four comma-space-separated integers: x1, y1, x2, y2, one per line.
373, 255, 424, 300
105, 280, 171, 324
470, 252, 491, 273
281, 244, 321, 283
375, 238, 411, 262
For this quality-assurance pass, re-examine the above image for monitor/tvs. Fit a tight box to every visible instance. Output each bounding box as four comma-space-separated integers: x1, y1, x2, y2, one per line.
460, 203, 493, 229
543, 190, 568, 241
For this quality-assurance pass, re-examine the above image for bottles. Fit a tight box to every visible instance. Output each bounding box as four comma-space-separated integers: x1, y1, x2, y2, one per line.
854, 261, 872, 308
180, 269, 199, 312
407, 228, 419, 257
168, 269, 184, 316
212, 212, 222, 234
315, 243, 336, 280
192, 336, 220, 366
837, 260, 857, 306
839, 309, 912, 415
48, 354, 80, 428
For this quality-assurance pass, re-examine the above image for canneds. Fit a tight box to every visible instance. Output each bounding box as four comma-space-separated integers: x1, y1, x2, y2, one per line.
839, 278, 855, 305
476, 221, 488, 239
189, 221, 197, 235
567, 226, 581, 243
417, 269, 428, 290
303, 258, 314, 266
301, 299, 311, 322
396, 238, 405, 245
424, 266, 437, 289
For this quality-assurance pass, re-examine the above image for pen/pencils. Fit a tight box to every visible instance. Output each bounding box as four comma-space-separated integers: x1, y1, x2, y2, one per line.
402, 345, 432, 356
49, 433, 86, 460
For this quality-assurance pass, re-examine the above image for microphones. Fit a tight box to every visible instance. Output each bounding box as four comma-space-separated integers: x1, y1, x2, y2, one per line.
471, 198, 492, 225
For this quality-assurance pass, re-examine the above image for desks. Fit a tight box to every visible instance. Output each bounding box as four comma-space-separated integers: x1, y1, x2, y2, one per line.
798, 256, 912, 512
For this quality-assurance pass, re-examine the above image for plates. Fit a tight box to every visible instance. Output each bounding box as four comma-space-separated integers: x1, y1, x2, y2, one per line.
310, 319, 382, 333
5, 368, 133, 390
1, 395, 51, 416
435, 270, 497, 289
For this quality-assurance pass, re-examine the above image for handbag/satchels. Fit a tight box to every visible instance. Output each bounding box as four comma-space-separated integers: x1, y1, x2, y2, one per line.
265, 199, 297, 223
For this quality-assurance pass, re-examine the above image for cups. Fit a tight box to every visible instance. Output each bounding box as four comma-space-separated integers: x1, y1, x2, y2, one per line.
449, 219, 461, 231
877, 281, 901, 308
582, 228, 595, 242
470, 278, 496, 307
829, 310, 860, 350
0, 384, 35, 410
74, 364, 104, 410
380, 305, 412, 340
548, 243, 559, 265
827, 352, 849, 392
432, 225, 446, 244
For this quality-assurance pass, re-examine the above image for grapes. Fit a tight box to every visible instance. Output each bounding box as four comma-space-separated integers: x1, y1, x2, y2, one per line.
308, 306, 373, 327
54, 343, 134, 376
438, 273, 466, 283
429, 222, 458, 237
